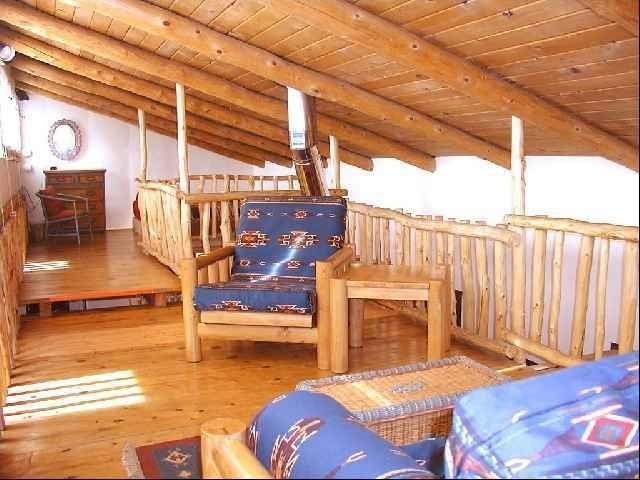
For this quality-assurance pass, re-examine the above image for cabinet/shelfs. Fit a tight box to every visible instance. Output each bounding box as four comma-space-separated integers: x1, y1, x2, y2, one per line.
42, 169, 108, 233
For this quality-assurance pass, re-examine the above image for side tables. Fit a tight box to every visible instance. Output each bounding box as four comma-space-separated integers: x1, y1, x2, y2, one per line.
330, 262, 450, 372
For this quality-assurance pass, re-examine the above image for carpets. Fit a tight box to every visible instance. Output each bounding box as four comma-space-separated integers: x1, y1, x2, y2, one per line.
123, 436, 204, 479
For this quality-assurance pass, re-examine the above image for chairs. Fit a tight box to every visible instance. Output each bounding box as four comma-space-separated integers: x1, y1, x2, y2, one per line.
181, 194, 348, 370
213, 350, 639, 479
35, 191, 93, 246
